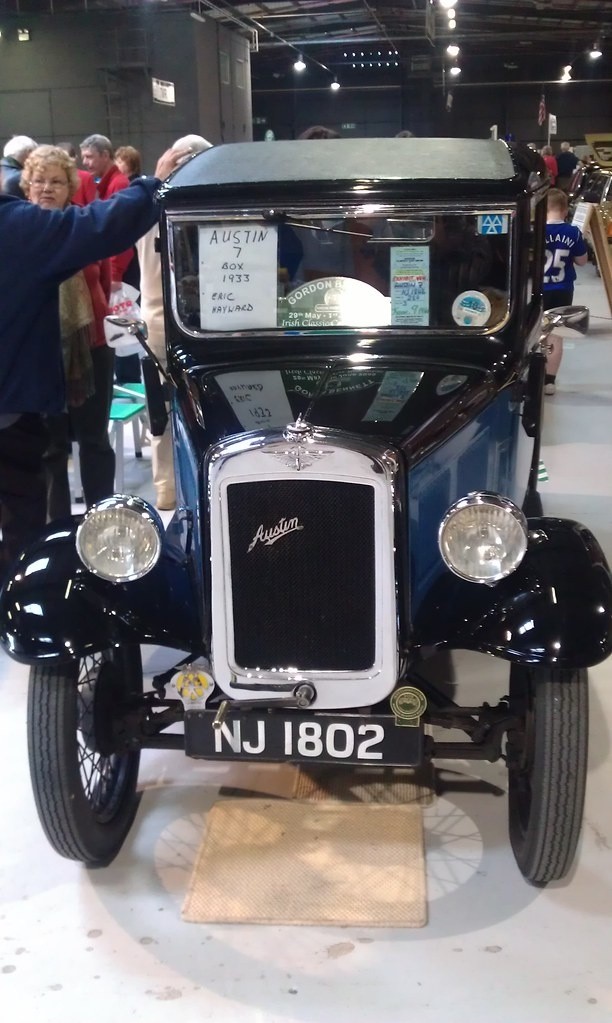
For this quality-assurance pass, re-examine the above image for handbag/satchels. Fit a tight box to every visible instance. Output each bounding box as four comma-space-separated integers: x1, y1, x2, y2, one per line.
109, 282, 141, 319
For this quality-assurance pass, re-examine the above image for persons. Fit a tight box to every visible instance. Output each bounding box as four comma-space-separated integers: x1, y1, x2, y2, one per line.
528, 142, 584, 192
0, 130, 209, 589
538, 188, 588, 395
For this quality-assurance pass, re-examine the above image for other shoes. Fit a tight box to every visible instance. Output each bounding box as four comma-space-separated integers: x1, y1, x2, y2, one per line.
157, 502, 177, 510
544, 383, 555, 395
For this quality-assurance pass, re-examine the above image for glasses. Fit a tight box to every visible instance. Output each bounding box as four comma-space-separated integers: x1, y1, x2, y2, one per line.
28, 178, 67, 190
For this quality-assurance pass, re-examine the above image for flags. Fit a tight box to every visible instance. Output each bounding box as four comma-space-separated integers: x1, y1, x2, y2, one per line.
539, 98, 546, 126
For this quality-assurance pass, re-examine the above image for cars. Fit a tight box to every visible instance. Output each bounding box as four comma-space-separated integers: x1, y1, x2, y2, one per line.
0, 137, 611, 889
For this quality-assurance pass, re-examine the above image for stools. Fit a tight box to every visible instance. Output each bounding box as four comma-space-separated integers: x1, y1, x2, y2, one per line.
68, 380, 154, 504
